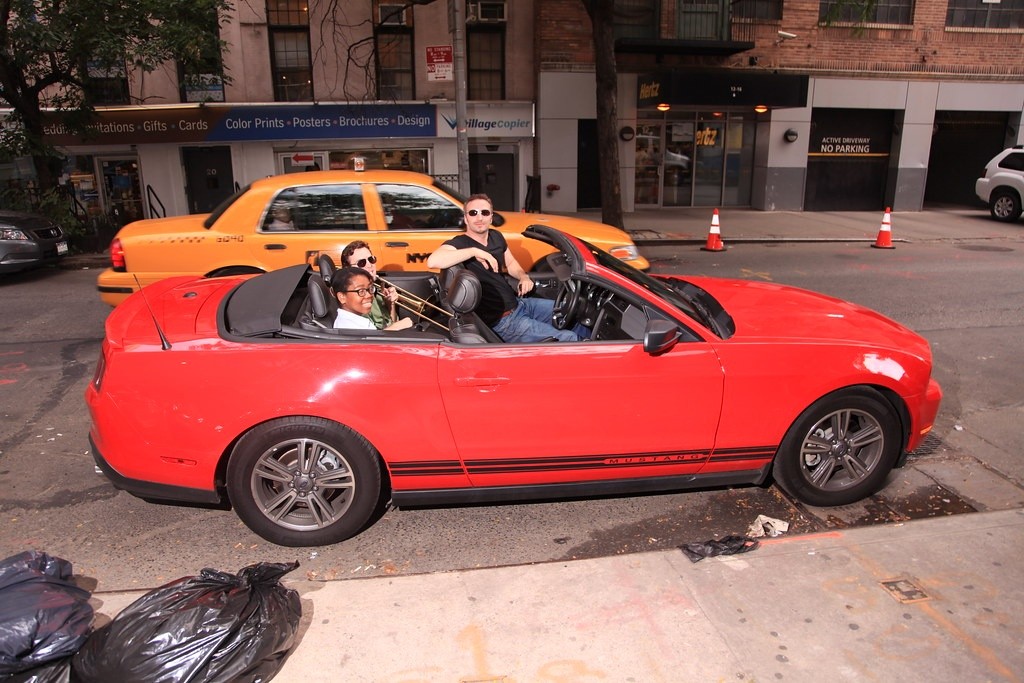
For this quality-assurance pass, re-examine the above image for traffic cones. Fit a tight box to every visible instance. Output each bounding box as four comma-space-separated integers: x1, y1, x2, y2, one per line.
700, 208, 728, 252
870, 207, 896, 249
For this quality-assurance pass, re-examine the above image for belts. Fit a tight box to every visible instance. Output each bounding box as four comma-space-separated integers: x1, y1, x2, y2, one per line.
501, 311, 512, 319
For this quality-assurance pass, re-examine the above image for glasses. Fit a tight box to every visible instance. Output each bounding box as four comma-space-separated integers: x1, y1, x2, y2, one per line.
466, 209, 492, 217
350, 256, 377, 268
340, 285, 377, 297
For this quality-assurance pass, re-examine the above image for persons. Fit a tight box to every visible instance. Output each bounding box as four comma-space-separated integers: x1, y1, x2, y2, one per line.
341, 241, 398, 330
113, 167, 131, 190
268, 200, 299, 231
427, 193, 591, 343
330, 268, 413, 331
636, 144, 649, 199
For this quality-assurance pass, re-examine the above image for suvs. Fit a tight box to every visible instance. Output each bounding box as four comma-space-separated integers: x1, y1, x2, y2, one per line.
636, 134, 694, 186
975, 144, 1024, 221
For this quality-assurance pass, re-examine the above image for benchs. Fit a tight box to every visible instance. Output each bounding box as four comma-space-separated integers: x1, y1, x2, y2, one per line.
307, 275, 333, 328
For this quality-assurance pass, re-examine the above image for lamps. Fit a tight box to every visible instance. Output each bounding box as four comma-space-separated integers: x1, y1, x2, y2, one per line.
785, 128, 798, 142
754, 105, 768, 113
619, 126, 635, 141
657, 104, 671, 111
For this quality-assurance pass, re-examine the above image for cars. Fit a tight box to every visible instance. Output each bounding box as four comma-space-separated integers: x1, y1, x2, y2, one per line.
84, 225, 942, 548
0, 207, 71, 283
96, 157, 653, 310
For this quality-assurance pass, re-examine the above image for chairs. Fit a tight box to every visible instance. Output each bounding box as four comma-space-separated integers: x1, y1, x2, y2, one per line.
438, 265, 506, 344
318, 254, 339, 307
